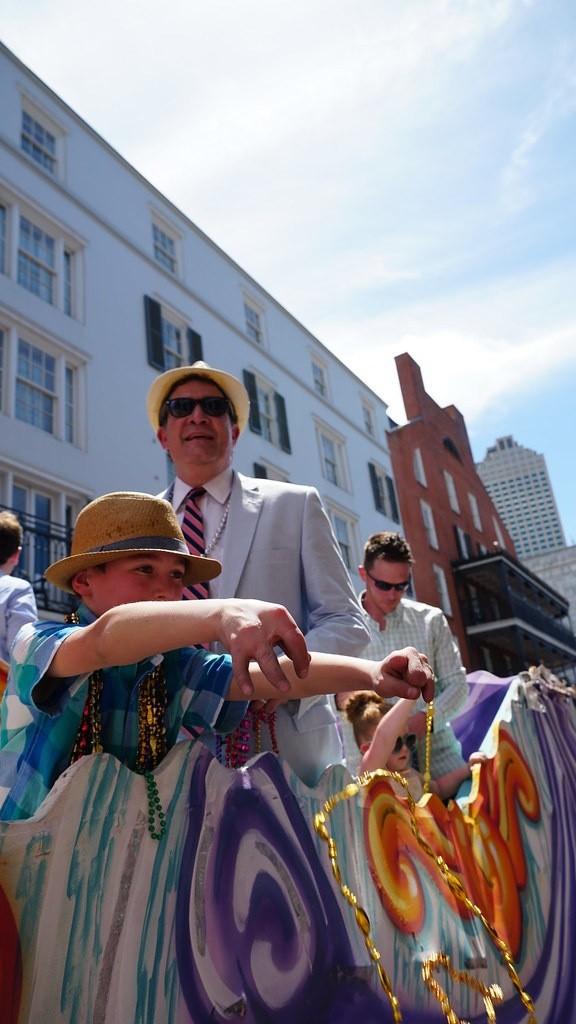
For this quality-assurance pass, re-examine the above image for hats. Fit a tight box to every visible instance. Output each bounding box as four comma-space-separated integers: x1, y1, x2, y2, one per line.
147, 361, 250, 432
44, 492, 222, 595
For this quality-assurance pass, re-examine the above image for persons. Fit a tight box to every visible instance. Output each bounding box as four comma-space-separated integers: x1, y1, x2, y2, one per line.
0, 492, 437, 822
150, 360, 371, 787
0, 510, 37, 710
347, 691, 488, 804
335, 532, 470, 800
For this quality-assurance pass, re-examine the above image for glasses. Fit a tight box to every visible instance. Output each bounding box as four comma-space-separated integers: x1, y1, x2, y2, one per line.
366, 569, 409, 592
166, 396, 228, 417
360, 733, 416, 754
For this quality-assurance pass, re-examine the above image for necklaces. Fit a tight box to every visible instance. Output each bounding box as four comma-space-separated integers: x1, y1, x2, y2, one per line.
314, 701, 535, 1024
200, 499, 232, 559
214, 703, 279, 769
66, 613, 168, 840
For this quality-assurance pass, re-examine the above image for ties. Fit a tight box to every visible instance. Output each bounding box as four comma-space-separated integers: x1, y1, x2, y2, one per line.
179, 488, 211, 744
